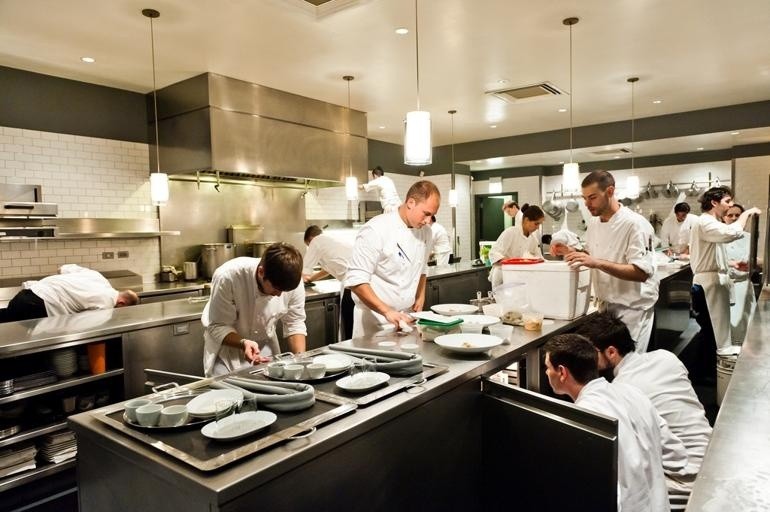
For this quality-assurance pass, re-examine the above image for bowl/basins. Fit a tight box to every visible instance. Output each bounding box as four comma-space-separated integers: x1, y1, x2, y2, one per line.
489, 324, 513, 344
522, 312, 544, 331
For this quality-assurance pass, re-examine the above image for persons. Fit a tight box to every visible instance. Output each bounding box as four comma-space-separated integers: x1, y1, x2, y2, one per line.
486, 201, 582, 289
543, 312, 714, 512
300, 225, 358, 340
351, 180, 441, 340
661, 186, 763, 351
200, 242, 307, 378
3, 263, 138, 323
429, 215, 452, 265
549, 169, 661, 354
358, 166, 402, 214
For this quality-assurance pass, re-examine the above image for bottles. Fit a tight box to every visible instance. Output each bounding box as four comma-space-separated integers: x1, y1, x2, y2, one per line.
471, 297, 495, 315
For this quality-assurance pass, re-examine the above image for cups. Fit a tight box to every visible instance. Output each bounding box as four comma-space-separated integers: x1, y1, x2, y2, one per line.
86, 342, 107, 375
213, 390, 258, 435
671, 252, 680, 261
267, 361, 326, 380
348, 355, 376, 385
183, 261, 199, 281
125, 398, 188, 426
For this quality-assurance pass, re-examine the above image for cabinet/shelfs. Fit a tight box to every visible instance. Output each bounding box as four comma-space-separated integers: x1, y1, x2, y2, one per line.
0, 367, 124, 493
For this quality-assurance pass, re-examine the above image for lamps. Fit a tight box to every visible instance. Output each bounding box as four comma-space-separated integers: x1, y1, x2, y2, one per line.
561, 16, 581, 192
626, 76, 641, 200
142, 7, 170, 207
446, 110, 461, 207
401, 0, 433, 168
341, 74, 359, 201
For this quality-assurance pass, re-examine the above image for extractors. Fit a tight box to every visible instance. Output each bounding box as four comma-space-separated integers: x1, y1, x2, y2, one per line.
144, 71, 369, 196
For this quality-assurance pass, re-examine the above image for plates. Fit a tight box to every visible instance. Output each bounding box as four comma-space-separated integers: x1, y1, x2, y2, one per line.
52, 349, 77, 378
313, 354, 354, 372
333, 371, 391, 391
427, 302, 504, 354
0, 378, 16, 395
186, 389, 245, 417
201, 410, 277, 441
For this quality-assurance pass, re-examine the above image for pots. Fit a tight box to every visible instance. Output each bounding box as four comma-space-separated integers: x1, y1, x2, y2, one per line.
541, 180, 701, 220
200, 242, 236, 280
246, 241, 277, 260
